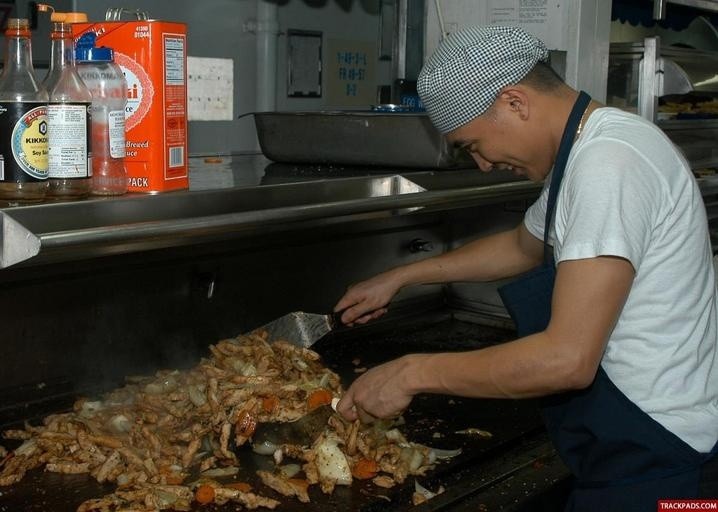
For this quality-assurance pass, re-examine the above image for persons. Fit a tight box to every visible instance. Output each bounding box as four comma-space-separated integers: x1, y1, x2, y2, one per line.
327, 23, 718, 511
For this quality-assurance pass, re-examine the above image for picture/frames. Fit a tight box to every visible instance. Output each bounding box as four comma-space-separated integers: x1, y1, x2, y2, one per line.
286, 28, 323, 98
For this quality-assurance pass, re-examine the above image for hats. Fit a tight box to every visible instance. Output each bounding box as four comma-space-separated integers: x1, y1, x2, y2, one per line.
417, 26, 548, 136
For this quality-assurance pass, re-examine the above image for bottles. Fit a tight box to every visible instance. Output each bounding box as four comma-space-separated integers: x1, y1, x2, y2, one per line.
41, 21, 94, 198
74, 47, 128, 197
0, 18, 50, 202
